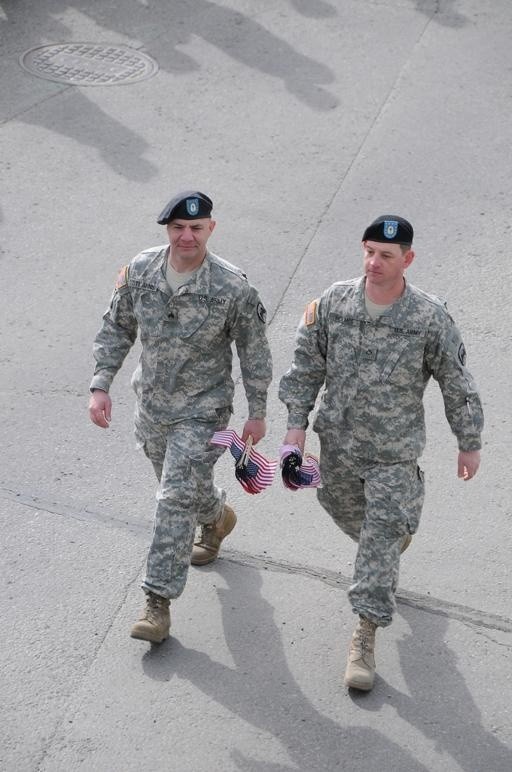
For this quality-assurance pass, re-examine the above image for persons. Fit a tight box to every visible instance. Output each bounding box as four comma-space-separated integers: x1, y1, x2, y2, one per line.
86, 190, 275, 646
275, 215, 485, 694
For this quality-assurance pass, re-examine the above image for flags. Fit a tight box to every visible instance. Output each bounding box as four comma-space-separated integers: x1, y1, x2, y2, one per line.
210, 428, 246, 463
234, 445, 323, 495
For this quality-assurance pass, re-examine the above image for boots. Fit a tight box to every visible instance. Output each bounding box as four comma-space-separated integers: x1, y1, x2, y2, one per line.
188, 503, 238, 566
343, 613, 378, 692
129, 586, 172, 644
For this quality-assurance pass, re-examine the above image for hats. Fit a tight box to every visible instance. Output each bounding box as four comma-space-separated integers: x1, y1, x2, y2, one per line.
360, 213, 414, 247
156, 190, 214, 227
